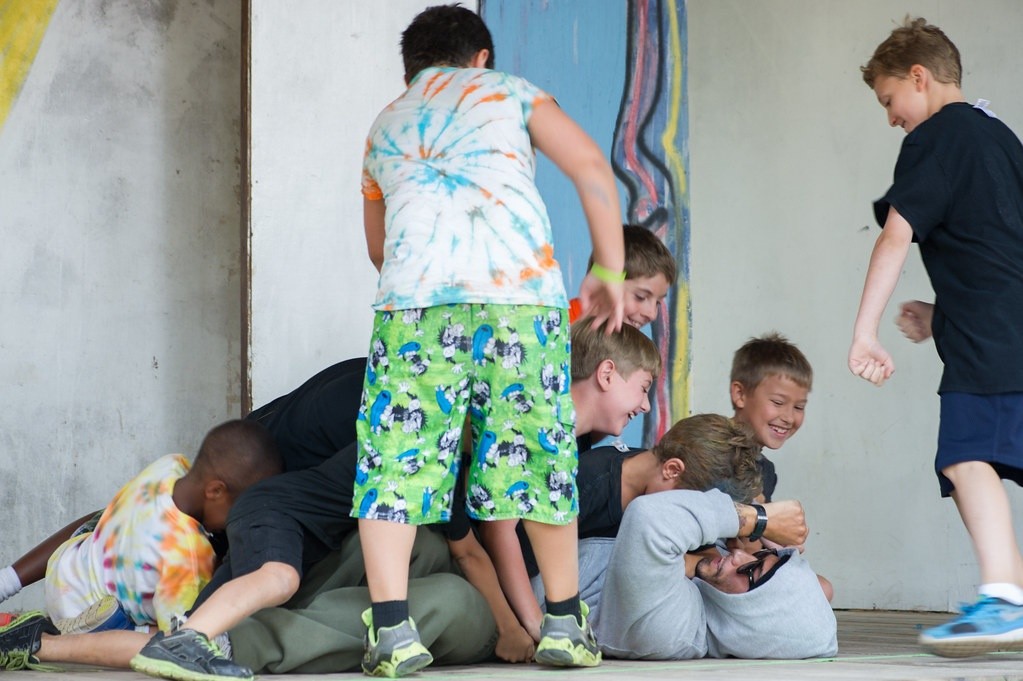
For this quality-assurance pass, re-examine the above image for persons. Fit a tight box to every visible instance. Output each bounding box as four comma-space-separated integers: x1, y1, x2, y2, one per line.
564, 222, 670, 331
338, 2, 631, 680
841, 9, 1023, 653
0, 313, 840, 681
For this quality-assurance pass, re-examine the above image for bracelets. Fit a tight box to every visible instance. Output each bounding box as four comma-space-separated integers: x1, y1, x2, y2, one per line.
588, 262, 629, 286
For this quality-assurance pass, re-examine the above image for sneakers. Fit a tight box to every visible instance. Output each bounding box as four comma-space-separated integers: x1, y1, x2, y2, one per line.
57, 596, 135, 633
0, 610, 63, 673
535, 602, 602, 668
920, 592, 1023, 657
361, 608, 433, 676
131, 629, 254, 681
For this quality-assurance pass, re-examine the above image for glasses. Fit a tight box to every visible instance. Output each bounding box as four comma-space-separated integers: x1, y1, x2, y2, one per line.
736, 547, 778, 592
